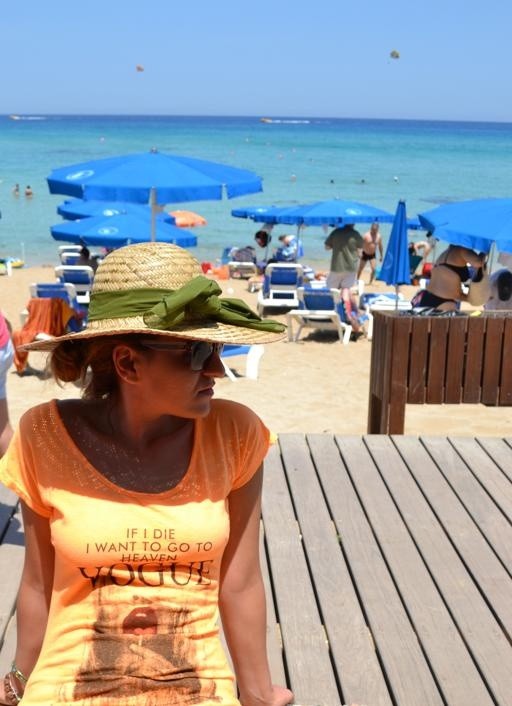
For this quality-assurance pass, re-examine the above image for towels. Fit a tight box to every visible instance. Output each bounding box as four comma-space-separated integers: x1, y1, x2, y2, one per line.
11, 297, 85, 374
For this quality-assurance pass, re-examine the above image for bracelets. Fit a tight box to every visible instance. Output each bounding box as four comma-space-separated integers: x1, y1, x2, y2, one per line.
4, 672, 20, 705
12, 661, 27, 684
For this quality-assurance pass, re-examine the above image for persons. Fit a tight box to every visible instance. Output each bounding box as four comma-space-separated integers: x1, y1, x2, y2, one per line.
75, 247, 98, 276
0, 311, 15, 458
0, 242, 295, 706
288, 172, 398, 186
13, 184, 33, 198
229, 223, 511, 340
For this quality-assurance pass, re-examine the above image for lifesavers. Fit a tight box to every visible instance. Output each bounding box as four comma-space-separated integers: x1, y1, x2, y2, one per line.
0, 257, 23, 275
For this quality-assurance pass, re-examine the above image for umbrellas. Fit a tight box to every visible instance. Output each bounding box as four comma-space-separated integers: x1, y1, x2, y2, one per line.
44, 147, 264, 246
418, 198, 512, 275
409, 217, 429, 231
232, 199, 397, 261
376, 198, 411, 311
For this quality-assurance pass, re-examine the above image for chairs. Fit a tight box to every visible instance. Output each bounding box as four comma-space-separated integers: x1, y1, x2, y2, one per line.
30, 283, 87, 331
359, 292, 413, 340
288, 287, 363, 343
219, 339, 263, 380
303, 265, 314, 280
249, 275, 364, 295
257, 263, 300, 315
19, 300, 73, 341
51, 244, 107, 302
228, 260, 254, 278
376, 256, 422, 282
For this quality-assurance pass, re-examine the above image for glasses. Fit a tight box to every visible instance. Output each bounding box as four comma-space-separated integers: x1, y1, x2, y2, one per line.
140, 342, 224, 372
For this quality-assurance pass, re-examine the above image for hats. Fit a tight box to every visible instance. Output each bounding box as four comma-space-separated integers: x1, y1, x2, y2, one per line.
14, 241, 288, 352
